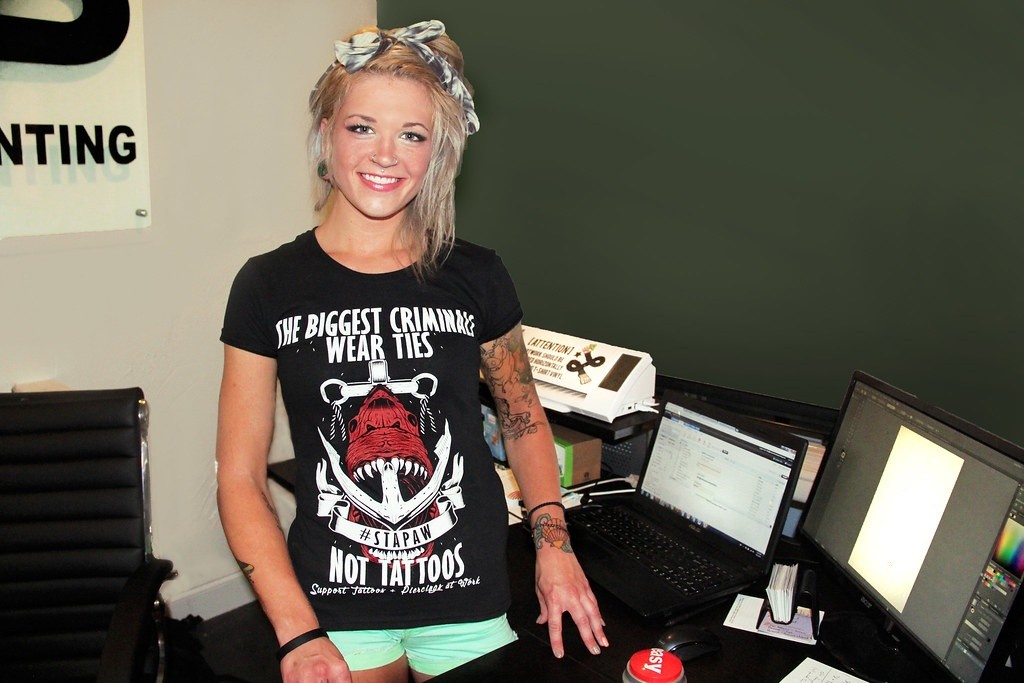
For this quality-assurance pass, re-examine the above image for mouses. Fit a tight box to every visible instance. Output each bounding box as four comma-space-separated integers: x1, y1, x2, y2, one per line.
657, 623, 721, 661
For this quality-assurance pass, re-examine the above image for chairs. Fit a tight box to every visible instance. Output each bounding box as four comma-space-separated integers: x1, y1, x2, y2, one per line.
0, 386, 177, 683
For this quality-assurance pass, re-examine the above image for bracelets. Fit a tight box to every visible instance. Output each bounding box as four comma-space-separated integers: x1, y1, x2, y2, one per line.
278, 627, 328, 663
528, 501, 569, 520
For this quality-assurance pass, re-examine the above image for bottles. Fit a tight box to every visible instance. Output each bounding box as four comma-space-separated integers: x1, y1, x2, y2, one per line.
622, 649, 688, 683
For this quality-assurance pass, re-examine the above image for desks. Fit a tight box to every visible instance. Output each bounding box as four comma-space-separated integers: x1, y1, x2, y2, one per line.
264, 431, 1024, 681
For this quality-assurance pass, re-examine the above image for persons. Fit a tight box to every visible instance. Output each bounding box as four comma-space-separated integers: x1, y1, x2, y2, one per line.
217, 20, 609, 683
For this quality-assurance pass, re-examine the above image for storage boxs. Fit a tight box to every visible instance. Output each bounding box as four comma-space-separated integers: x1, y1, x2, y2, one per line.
548, 422, 602, 488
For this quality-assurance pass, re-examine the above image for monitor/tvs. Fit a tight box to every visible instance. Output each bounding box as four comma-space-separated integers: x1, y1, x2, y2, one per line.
647, 369, 1024, 683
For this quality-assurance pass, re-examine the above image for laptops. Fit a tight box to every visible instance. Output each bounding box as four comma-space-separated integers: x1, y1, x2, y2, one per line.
523, 390, 809, 618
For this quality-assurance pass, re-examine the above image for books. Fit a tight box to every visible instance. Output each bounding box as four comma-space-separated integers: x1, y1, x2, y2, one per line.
765, 562, 799, 624
